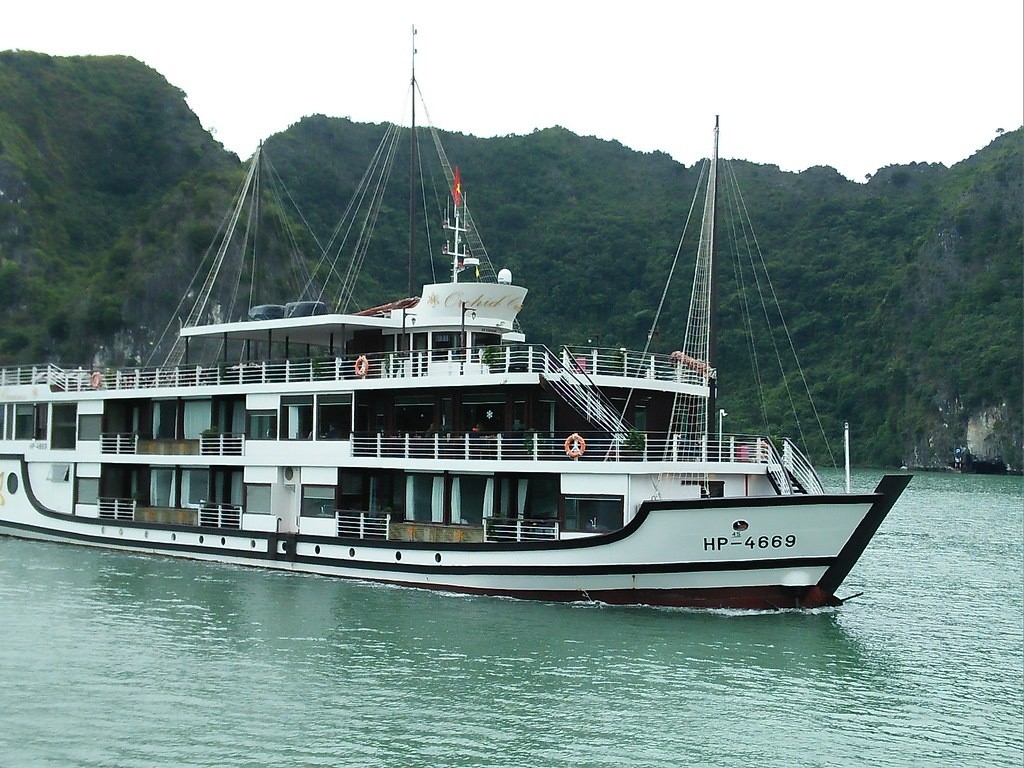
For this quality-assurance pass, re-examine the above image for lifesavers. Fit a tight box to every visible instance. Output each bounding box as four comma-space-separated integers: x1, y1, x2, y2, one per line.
564, 433, 586, 458
354, 355, 368, 377
90, 371, 101, 388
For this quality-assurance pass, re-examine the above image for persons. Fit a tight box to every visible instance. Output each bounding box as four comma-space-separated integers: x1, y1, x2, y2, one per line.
424, 424, 434, 442
470, 426, 479, 443
307, 424, 313, 439
615, 347, 631, 372
326, 422, 337, 437
754, 439, 767, 463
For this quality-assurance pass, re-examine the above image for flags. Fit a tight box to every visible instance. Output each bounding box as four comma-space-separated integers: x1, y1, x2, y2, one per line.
452, 166, 460, 205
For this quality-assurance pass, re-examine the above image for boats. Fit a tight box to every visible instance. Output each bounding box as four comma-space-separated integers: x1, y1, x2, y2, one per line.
900, 448, 1007, 476
1, 25, 912, 612
